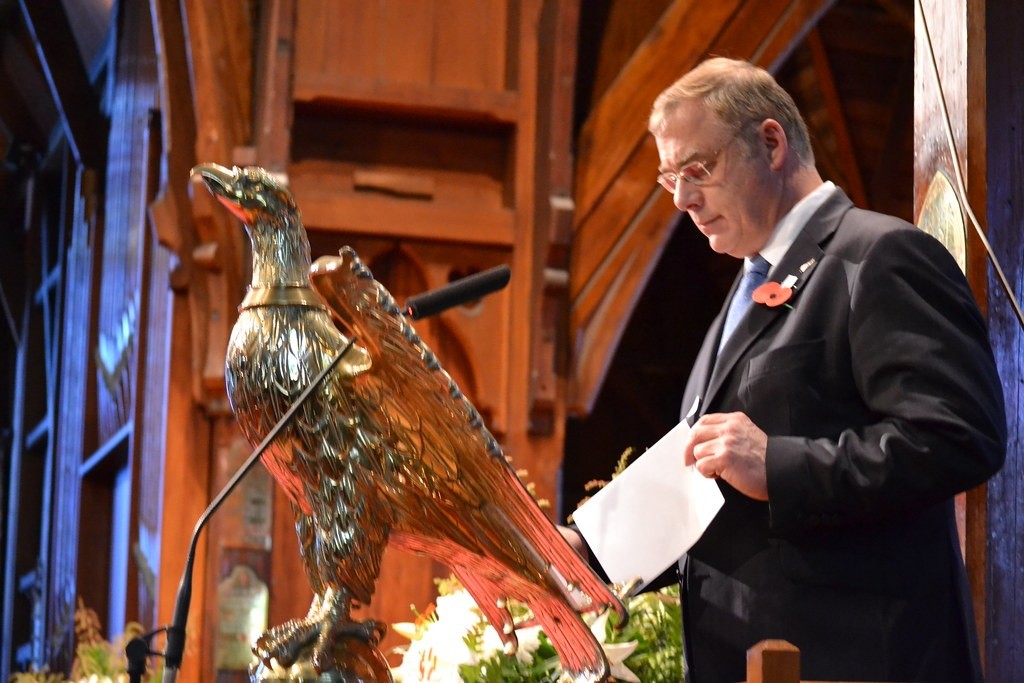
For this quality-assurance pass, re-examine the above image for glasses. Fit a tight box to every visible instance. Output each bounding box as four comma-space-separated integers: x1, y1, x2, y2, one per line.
656, 123, 747, 193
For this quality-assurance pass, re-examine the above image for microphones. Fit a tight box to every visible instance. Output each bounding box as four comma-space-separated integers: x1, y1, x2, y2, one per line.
160, 263, 512, 683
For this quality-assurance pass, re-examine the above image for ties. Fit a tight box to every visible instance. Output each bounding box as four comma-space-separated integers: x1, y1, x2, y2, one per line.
717, 256, 770, 371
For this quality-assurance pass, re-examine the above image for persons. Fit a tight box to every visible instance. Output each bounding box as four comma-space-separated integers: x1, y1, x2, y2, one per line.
551, 57, 1007, 683
